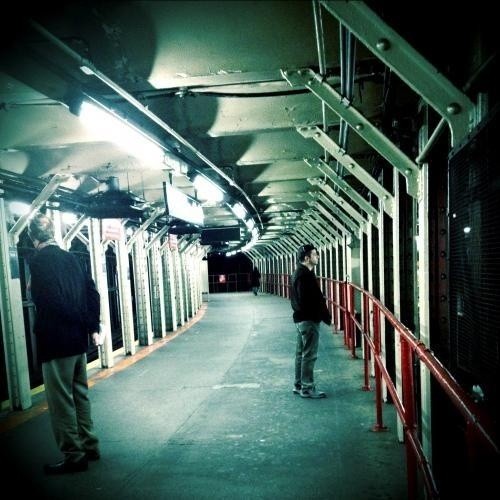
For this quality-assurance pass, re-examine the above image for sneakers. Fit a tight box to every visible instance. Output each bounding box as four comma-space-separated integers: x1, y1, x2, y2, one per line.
56, 449, 100, 462
292, 386, 300, 394
300, 389, 328, 399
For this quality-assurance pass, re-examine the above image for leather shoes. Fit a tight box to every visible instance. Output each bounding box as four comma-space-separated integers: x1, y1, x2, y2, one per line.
43, 458, 91, 473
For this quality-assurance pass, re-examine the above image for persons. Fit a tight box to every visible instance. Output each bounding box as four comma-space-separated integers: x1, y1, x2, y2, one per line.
249, 267, 260, 296
289, 244, 331, 400
26, 213, 100, 476
